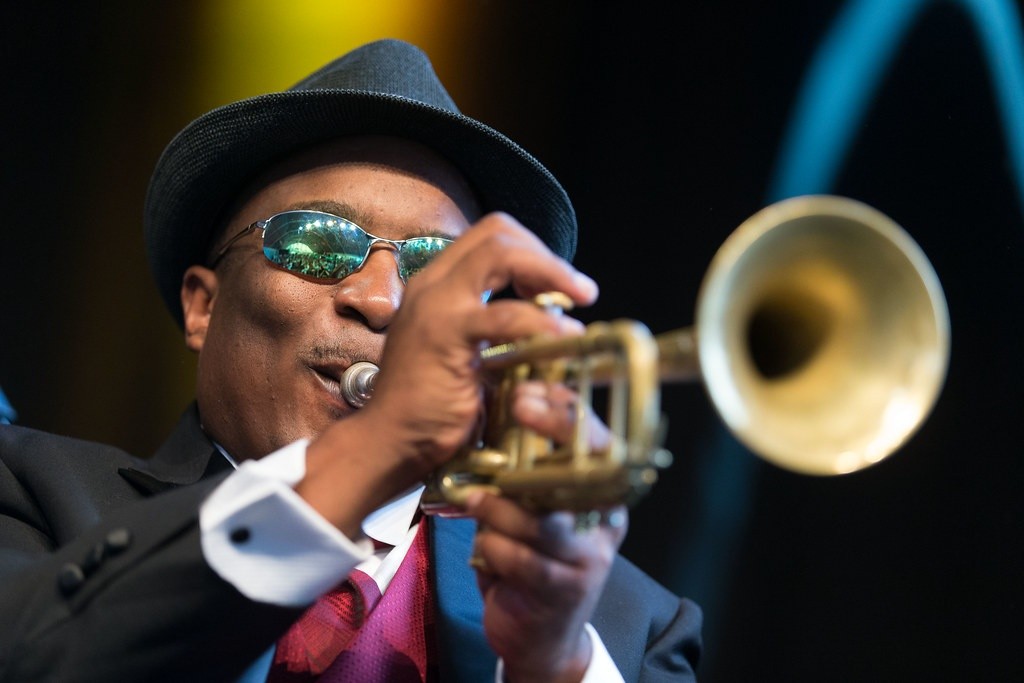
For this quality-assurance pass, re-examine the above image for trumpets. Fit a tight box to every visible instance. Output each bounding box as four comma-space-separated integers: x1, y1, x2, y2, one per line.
336, 192, 952, 506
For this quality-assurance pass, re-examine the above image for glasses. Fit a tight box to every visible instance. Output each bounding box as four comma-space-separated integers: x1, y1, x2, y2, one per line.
208, 209, 492, 303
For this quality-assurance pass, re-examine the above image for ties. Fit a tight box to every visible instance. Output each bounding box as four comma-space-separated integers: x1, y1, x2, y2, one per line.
262, 515, 429, 683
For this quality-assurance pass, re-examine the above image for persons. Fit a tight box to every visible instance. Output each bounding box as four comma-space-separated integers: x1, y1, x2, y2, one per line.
0, 36, 703, 682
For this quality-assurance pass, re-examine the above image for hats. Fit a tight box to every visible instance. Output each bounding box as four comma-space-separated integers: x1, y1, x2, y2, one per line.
141, 39, 578, 333
